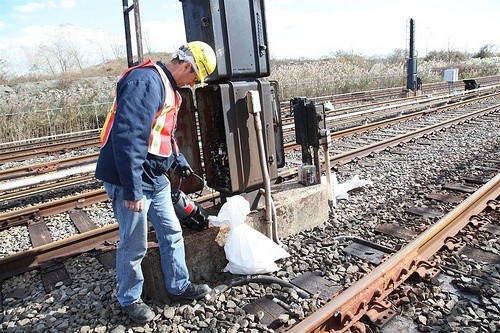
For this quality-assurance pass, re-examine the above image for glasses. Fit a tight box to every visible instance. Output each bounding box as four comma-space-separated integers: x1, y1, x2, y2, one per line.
191, 70, 201, 85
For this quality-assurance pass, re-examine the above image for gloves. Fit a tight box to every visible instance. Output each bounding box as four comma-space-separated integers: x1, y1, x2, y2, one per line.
170, 153, 194, 178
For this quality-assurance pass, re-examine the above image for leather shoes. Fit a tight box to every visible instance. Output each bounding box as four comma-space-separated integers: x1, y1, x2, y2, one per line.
122, 299, 155, 323
169, 282, 212, 302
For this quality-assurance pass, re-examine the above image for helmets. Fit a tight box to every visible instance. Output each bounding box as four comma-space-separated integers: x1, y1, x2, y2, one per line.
177, 41, 216, 88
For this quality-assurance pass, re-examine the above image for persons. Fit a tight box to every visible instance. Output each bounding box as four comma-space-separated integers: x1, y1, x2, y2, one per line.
95, 41, 217, 324
